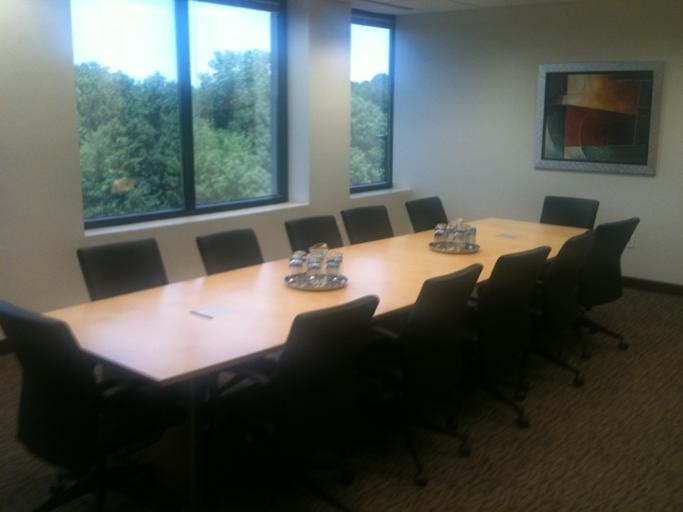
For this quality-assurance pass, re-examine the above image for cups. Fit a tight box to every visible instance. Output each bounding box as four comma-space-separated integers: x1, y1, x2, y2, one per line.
427, 217, 481, 255
282, 241, 350, 292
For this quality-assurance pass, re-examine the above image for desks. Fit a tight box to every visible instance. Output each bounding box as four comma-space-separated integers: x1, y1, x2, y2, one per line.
35, 217, 603, 509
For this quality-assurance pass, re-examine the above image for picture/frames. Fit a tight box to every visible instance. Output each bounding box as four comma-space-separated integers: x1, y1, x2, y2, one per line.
533, 58, 664, 177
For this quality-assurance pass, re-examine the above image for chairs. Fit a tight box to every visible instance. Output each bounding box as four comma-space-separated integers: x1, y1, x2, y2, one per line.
534, 195, 602, 227
1, 299, 146, 510
548, 214, 640, 361
